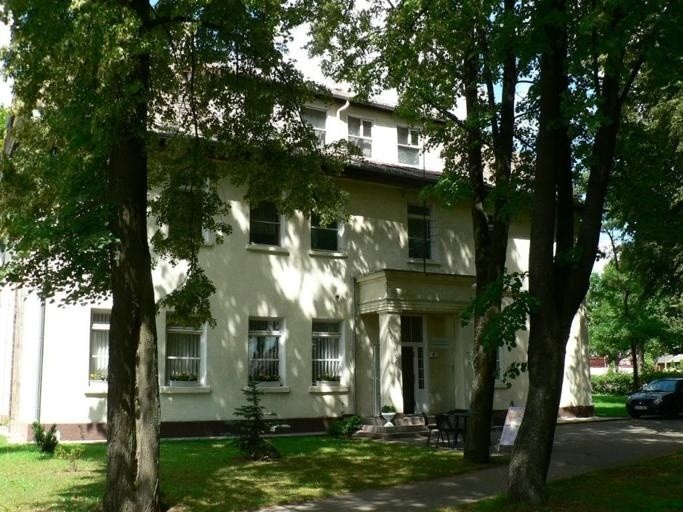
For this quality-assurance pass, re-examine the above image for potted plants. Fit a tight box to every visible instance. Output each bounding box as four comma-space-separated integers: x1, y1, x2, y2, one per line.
380, 405, 397, 427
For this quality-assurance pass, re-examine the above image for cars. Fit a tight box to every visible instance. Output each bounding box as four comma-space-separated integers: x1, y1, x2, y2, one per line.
624, 375, 682, 420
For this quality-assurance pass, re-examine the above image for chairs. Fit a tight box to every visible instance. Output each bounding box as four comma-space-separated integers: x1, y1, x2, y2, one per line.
422, 409, 468, 449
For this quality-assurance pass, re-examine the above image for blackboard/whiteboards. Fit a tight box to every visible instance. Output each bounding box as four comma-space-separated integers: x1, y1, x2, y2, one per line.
499, 407, 526, 446
